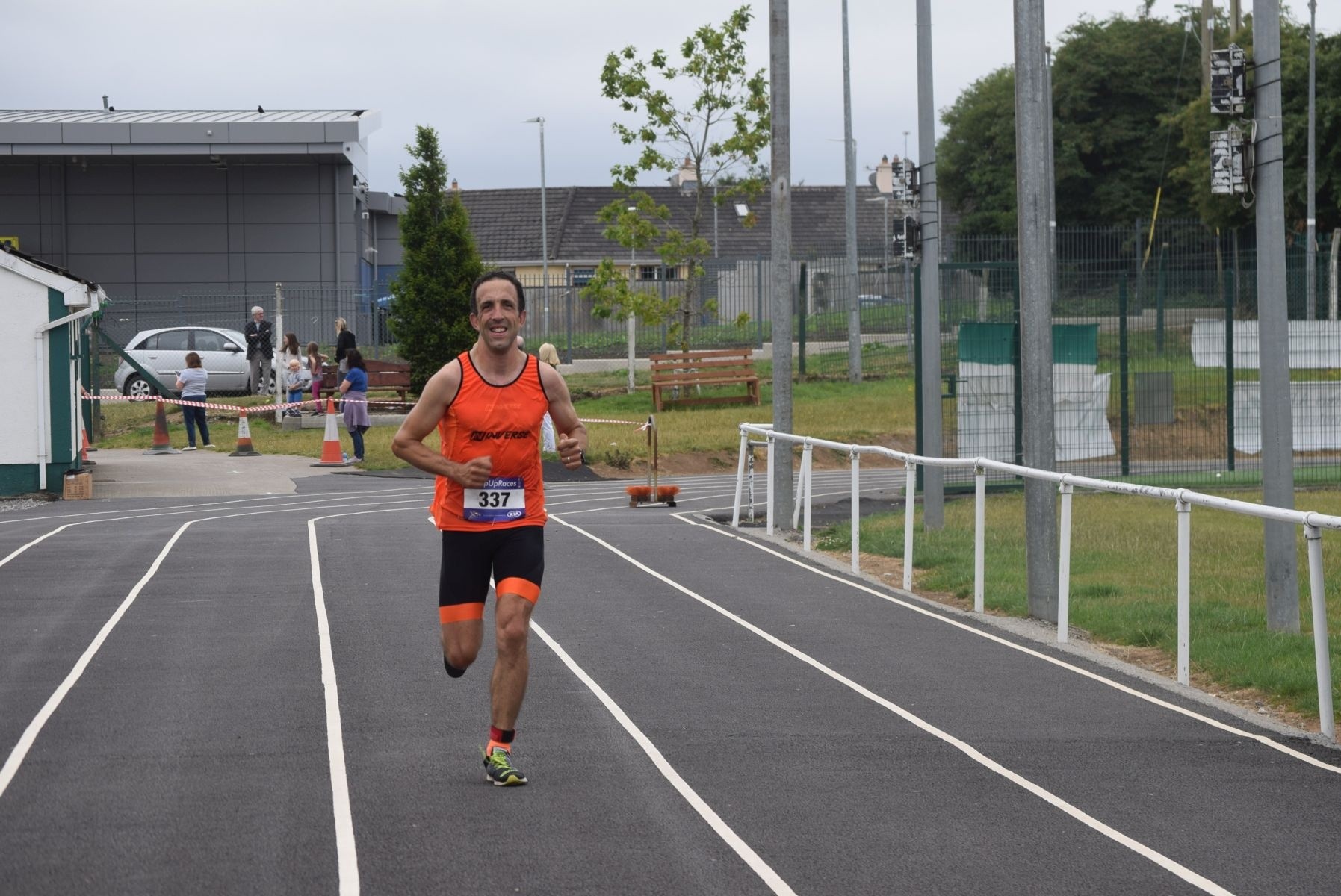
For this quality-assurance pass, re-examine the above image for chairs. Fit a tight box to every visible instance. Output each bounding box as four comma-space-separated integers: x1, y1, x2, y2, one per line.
207, 341, 217, 350
196, 339, 206, 351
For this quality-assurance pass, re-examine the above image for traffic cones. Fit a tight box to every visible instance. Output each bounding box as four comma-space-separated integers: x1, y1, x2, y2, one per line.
309, 396, 357, 467
81, 416, 99, 453
142, 399, 182, 455
80, 433, 98, 466
228, 406, 262, 457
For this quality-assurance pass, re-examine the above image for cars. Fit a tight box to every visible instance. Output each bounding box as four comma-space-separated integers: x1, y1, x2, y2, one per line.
114, 326, 334, 402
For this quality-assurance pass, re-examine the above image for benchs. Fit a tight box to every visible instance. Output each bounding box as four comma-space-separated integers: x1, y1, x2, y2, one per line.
319, 359, 412, 415
650, 348, 760, 413
158, 339, 182, 349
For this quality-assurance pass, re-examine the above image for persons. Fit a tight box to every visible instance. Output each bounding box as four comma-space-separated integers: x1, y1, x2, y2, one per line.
391, 269, 588, 787
334, 317, 358, 414
244, 306, 274, 394
538, 342, 560, 454
307, 341, 329, 417
286, 358, 305, 417
339, 349, 370, 464
174, 352, 215, 451
517, 335, 525, 352
279, 333, 302, 417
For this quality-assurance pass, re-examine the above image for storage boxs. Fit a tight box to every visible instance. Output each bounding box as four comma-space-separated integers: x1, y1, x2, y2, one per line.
63, 472, 93, 500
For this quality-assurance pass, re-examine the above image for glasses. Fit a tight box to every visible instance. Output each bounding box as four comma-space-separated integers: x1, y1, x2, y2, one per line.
256, 312, 264, 315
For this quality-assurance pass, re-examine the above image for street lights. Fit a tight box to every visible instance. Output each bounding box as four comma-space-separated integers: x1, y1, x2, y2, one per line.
524, 115, 551, 344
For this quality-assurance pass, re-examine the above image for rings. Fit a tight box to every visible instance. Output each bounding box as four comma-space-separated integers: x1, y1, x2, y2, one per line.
567, 456, 570, 463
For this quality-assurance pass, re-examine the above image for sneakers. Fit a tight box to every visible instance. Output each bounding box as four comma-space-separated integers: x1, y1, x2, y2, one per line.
477, 743, 528, 784
311, 410, 324, 417
182, 446, 197, 451
343, 455, 362, 464
204, 444, 215, 448
